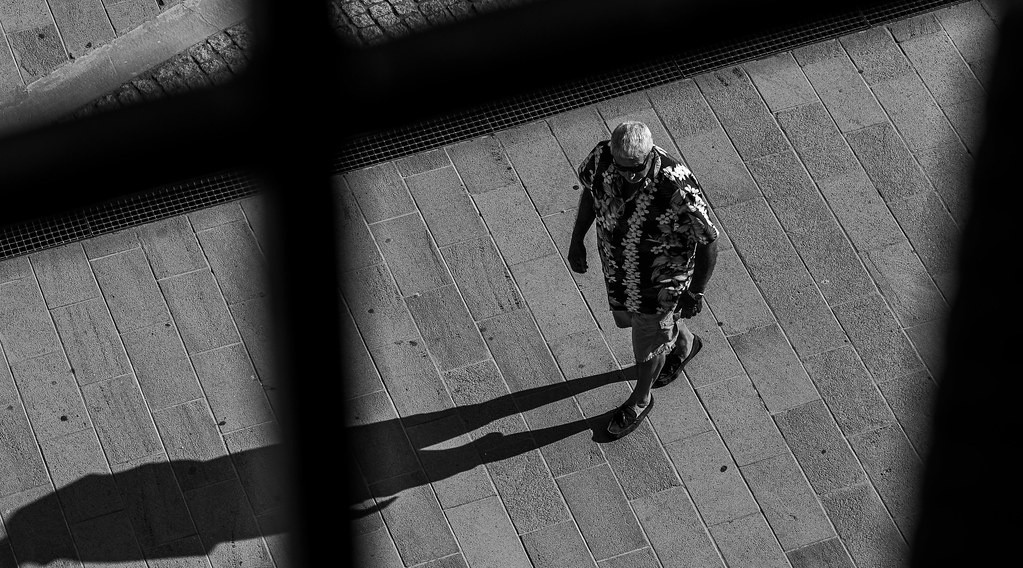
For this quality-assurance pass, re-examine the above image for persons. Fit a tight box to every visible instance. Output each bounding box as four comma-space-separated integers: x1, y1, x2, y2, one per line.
566, 121, 719, 439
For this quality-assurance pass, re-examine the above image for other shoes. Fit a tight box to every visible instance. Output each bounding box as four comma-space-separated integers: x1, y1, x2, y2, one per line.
653, 335, 702, 386
607, 394, 655, 438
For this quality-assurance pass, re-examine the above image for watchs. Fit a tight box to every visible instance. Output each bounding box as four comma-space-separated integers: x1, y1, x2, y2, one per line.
687, 289, 704, 301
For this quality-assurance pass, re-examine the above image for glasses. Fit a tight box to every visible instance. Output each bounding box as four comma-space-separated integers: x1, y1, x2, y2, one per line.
611, 148, 655, 173
